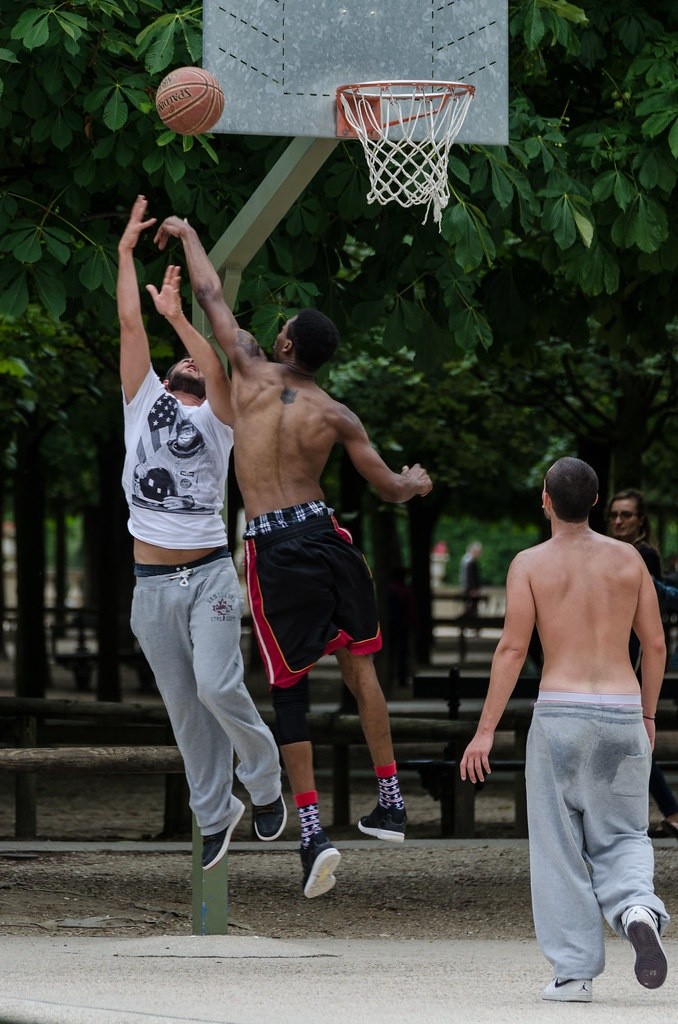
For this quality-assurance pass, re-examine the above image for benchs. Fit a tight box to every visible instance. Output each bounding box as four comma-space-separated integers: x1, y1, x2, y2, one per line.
396, 676, 678, 840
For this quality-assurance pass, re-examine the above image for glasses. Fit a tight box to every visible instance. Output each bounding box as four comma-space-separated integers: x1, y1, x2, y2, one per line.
608, 512, 638, 520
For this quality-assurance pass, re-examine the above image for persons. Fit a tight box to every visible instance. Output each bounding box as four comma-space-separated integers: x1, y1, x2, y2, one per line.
461, 543, 483, 636
608, 489, 678, 837
460, 457, 670, 1003
117, 193, 288, 870
153, 216, 434, 897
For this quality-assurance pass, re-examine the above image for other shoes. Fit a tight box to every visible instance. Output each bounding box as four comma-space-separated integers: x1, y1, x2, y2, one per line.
647, 820, 678, 838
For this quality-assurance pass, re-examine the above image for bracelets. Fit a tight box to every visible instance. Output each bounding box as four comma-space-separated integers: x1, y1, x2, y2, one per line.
643, 716, 656, 720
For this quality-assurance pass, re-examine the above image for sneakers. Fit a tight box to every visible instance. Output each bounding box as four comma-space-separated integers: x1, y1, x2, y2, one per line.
299, 831, 341, 898
201, 803, 246, 870
626, 906, 668, 989
358, 801, 407, 843
541, 978, 593, 1002
251, 792, 287, 841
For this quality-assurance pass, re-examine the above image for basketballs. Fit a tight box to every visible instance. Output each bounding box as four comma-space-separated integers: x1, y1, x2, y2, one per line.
156, 62, 226, 137
